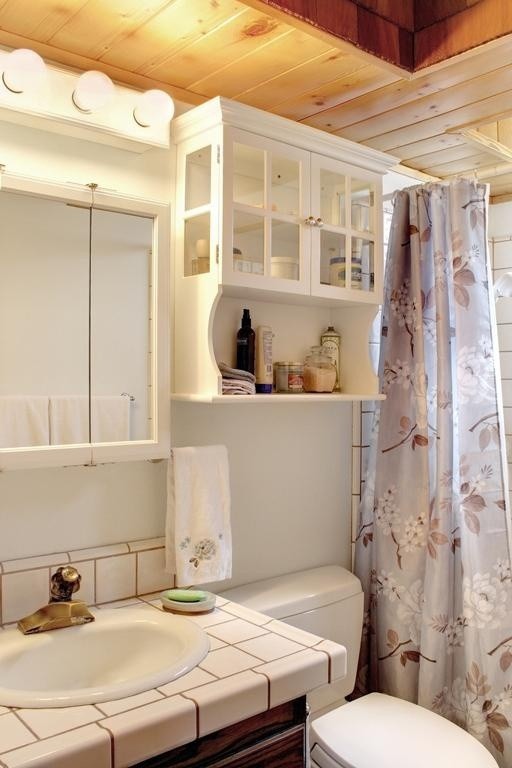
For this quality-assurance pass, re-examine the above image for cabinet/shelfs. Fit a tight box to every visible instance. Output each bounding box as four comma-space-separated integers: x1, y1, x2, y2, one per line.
122, 692, 310, 767
169, 98, 405, 403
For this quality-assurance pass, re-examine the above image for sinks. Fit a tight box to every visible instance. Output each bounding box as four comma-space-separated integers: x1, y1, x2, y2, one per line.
0, 606, 210, 708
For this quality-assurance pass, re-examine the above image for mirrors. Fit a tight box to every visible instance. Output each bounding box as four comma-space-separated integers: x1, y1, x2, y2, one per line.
0, 171, 169, 470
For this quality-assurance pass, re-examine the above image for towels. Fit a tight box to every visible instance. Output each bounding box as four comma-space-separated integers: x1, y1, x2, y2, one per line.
163, 439, 236, 587
0, 395, 132, 451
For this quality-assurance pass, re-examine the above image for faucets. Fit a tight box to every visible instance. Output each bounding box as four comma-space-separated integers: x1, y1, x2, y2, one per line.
17, 565, 95, 634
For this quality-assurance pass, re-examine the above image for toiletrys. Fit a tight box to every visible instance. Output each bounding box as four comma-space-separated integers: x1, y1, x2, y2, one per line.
321, 325, 342, 393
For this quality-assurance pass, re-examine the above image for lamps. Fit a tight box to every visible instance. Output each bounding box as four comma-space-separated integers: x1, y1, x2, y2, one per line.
2, 50, 172, 149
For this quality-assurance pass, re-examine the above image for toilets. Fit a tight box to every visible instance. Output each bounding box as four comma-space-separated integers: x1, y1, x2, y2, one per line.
215, 564, 499, 768
